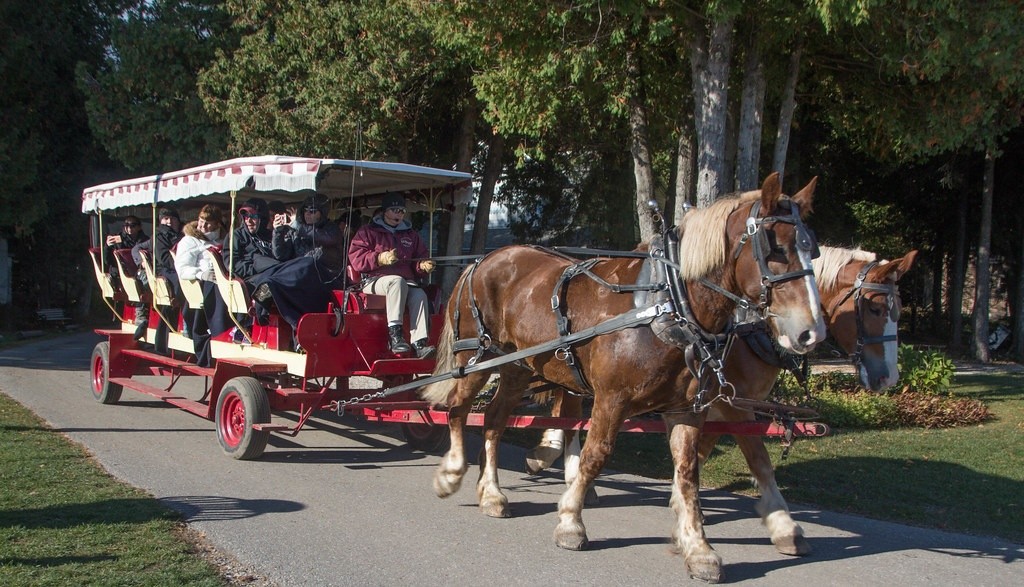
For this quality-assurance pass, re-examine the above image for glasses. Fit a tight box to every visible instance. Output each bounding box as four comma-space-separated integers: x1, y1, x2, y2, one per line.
123, 222, 140, 227
244, 212, 262, 220
304, 205, 322, 213
390, 208, 407, 214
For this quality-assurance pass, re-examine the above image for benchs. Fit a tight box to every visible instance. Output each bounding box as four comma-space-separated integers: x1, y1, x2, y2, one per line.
33, 307, 73, 328
90, 246, 439, 320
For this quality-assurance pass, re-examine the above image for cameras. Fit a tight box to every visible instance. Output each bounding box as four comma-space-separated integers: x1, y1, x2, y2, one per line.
280, 213, 288, 225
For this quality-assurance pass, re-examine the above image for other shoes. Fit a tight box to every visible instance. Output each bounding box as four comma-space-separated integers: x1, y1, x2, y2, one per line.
294, 339, 305, 355
135, 311, 148, 325
234, 329, 251, 345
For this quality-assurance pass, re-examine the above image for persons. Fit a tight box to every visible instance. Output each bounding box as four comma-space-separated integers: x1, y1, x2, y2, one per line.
348, 192, 438, 360
100, 195, 383, 369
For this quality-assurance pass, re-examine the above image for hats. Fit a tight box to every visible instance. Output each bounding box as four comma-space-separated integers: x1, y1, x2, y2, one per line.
381, 193, 406, 212
158, 205, 179, 222
238, 206, 258, 217
340, 209, 363, 235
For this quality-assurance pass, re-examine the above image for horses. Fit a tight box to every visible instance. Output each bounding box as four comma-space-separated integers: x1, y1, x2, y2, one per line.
416, 171, 827, 584
524, 245, 918, 508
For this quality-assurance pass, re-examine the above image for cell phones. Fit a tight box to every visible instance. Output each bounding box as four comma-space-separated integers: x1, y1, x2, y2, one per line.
111, 235, 122, 243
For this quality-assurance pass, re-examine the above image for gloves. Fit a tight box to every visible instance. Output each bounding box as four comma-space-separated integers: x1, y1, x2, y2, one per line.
419, 260, 436, 273
377, 248, 399, 266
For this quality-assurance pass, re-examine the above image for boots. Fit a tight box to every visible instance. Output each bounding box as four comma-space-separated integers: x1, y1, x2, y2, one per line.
388, 325, 410, 353
413, 338, 435, 359
